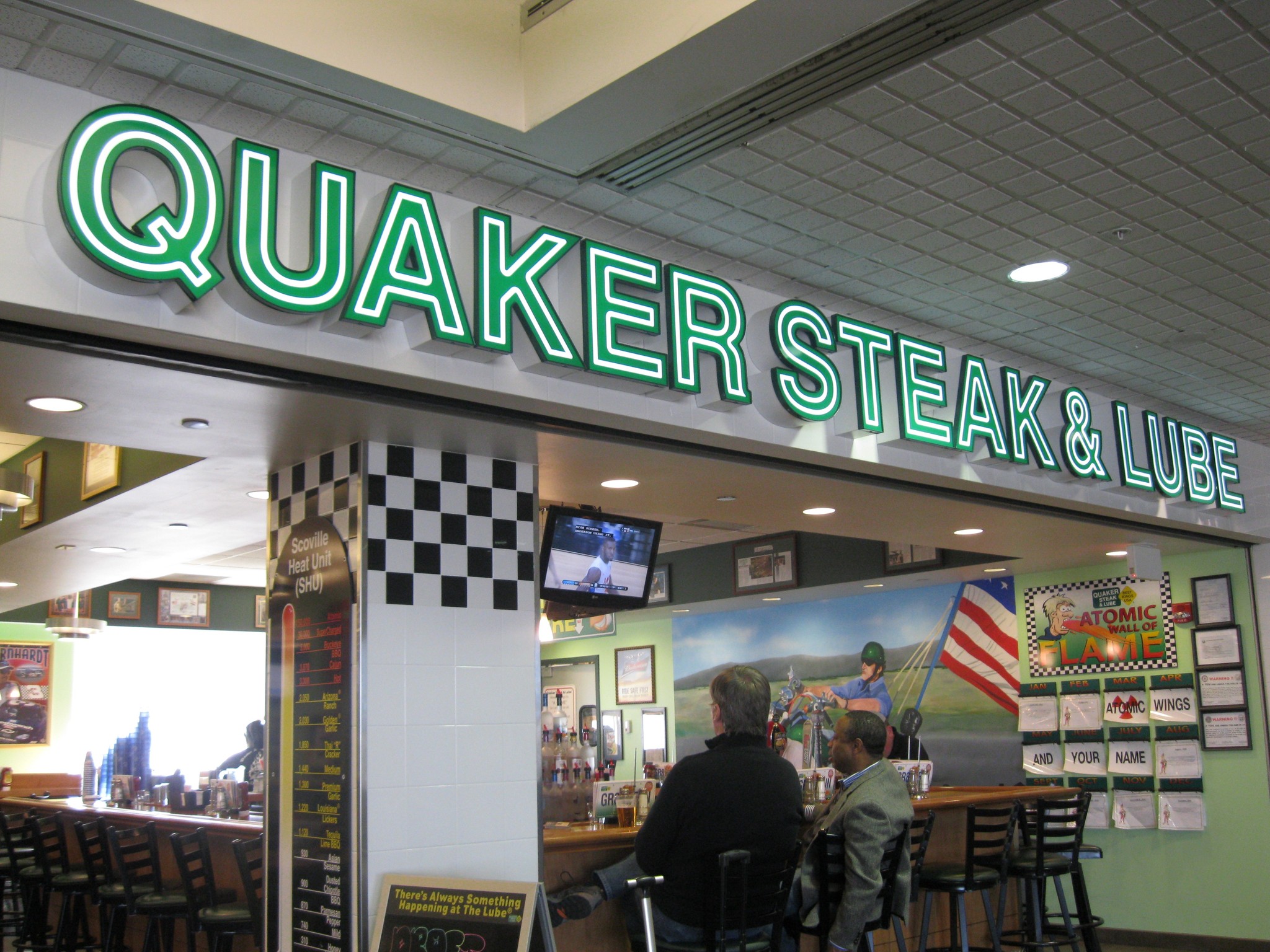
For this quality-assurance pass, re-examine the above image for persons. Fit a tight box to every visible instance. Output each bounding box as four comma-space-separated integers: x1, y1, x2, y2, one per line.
789, 641, 893, 726
209, 718, 266, 791
576, 537, 620, 594
541, 665, 812, 951
871, 710, 930, 762
775, 708, 917, 952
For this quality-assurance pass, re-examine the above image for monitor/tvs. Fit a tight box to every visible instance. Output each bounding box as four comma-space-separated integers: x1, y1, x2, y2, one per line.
541, 504, 665, 616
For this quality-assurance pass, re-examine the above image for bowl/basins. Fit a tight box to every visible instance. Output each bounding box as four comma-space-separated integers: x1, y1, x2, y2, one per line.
173, 790, 204, 810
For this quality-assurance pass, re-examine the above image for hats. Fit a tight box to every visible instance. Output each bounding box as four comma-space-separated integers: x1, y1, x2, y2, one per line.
0, 660, 14, 670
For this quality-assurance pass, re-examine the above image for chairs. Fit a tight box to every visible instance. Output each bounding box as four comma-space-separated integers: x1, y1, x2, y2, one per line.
703, 789, 1106, 952
1, 809, 265, 952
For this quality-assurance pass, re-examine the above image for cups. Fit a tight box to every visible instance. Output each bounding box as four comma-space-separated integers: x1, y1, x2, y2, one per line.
82, 710, 151, 803
153, 783, 170, 804
586, 801, 606, 824
615, 795, 637, 829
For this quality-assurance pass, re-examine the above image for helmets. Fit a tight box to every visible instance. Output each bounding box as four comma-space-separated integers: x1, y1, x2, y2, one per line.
861, 641, 886, 665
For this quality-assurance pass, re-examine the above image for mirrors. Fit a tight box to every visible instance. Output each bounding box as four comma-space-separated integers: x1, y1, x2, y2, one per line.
541, 654, 603, 773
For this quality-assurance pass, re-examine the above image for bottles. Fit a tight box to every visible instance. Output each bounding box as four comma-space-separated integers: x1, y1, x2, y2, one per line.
210, 785, 226, 810
620, 787, 648, 826
817, 775, 825, 801
541, 688, 616, 821
909, 768, 919, 795
920, 769, 930, 795
803, 777, 815, 805
2, 767, 12, 792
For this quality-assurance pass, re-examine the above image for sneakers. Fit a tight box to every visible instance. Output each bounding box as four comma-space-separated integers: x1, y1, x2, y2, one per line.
545, 883, 604, 928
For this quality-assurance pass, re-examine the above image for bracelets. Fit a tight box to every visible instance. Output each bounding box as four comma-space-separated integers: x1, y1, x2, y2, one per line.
841, 698, 849, 711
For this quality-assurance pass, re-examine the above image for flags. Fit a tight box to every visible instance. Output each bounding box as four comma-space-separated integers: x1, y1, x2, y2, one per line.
939, 576, 1020, 718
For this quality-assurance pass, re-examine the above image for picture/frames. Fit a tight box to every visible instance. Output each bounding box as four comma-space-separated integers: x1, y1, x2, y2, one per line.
80, 442, 120, 501
1186, 572, 1254, 752
600, 708, 624, 761
21, 451, 46, 529
640, 707, 667, 766
46, 585, 212, 630
614, 645, 655, 703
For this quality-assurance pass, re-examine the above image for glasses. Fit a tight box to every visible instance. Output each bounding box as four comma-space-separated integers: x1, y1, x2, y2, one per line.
861, 657, 876, 666
0, 669, 10, 675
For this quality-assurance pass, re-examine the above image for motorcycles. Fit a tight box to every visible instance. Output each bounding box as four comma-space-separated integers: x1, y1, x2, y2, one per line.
760, 676, 929, 779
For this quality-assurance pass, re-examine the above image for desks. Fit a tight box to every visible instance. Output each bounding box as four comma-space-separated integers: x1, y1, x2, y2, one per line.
0, 797, 261, 903
537, 774, 1083, 949
